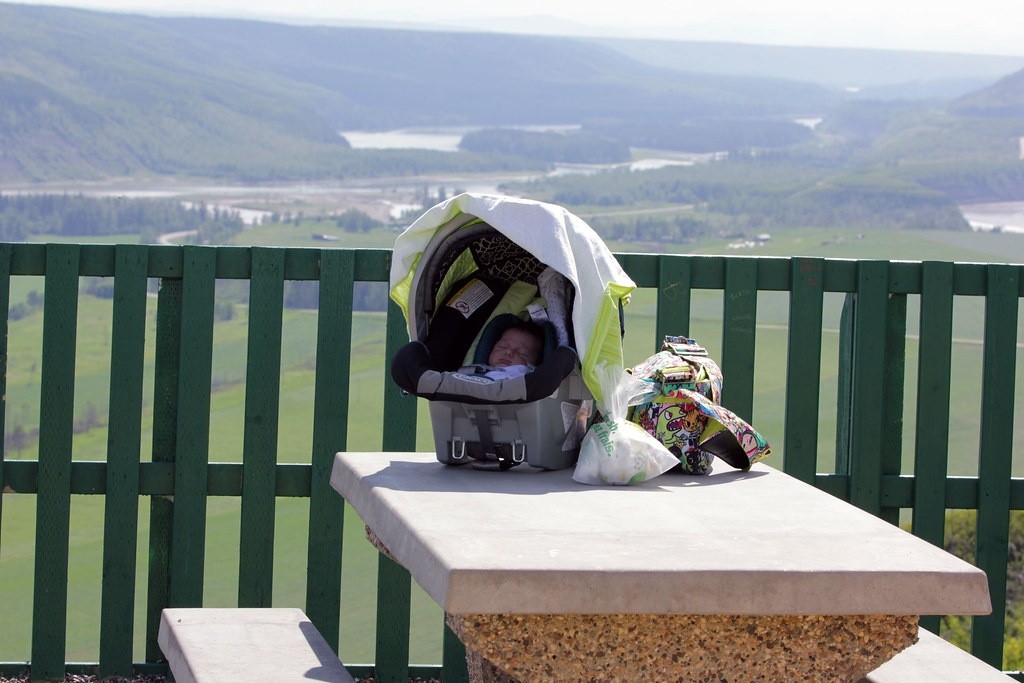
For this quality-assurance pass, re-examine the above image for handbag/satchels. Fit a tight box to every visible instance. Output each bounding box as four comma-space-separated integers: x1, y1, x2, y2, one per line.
621, 335, 772, 477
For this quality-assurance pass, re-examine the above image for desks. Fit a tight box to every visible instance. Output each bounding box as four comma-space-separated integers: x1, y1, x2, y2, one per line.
328, 452, 992, 683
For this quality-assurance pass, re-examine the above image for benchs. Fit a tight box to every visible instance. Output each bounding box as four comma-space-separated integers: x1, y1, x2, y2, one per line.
158, 608, 355, 683
865, 627, 1020, 683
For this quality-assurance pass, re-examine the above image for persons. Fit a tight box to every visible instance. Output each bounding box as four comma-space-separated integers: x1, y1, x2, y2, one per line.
483, 322, 545, 380
